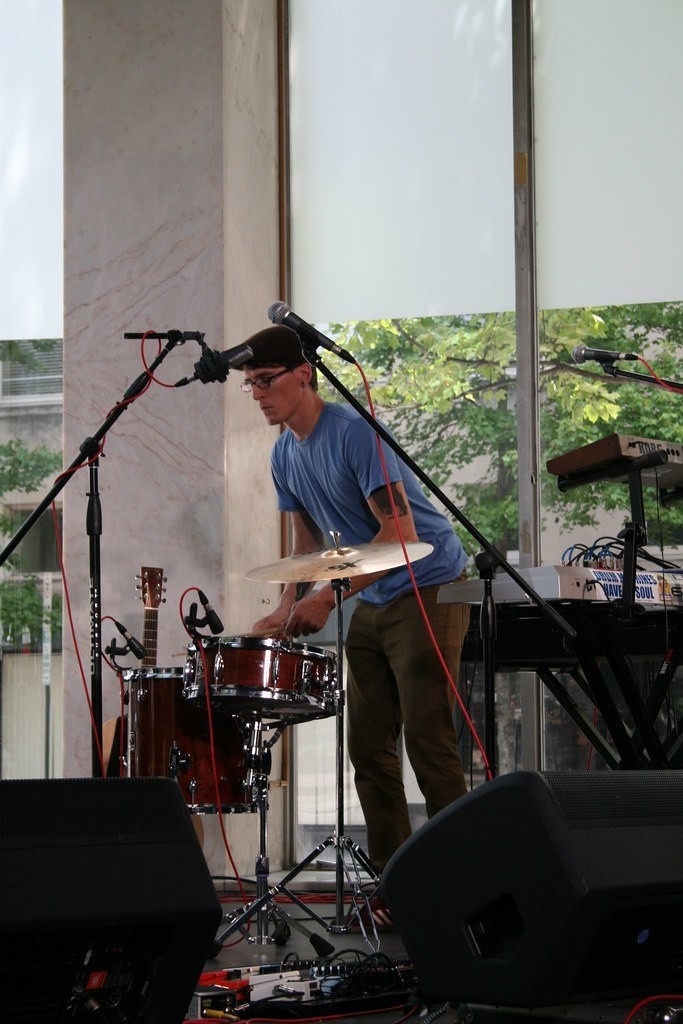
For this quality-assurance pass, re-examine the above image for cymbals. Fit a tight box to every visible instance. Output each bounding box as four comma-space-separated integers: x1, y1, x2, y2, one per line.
245, 541, 436, 588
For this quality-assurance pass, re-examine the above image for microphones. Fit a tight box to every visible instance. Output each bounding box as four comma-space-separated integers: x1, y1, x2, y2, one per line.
269, 301, 356, 362
174, 343, 253, 388
115, 621, 148, 660
198, 590, 224, 635
572, 344, 639, 365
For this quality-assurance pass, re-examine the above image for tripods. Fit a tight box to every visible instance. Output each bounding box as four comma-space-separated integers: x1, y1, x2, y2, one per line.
207, 579, 384, 962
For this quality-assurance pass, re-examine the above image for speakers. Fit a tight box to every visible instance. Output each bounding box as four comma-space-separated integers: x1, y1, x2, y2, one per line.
0, 778, 224, 1024
380, 769, 683, 1008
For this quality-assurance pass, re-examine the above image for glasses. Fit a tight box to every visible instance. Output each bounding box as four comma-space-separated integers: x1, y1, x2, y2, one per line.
239, 363, 305, 393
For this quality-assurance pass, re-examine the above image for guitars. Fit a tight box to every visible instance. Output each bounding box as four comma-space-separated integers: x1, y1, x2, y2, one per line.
91, 565, 208, 849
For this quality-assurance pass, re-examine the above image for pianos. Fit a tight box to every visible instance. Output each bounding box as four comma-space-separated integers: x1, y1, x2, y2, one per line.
544, 429, 683, 493
437, 558, 683, 660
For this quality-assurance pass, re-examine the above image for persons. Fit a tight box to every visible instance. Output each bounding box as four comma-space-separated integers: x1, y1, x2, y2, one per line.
229, 325, 472, 933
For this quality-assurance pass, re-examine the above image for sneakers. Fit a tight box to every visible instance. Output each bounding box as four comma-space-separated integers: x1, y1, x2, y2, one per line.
329, 896, 399, 933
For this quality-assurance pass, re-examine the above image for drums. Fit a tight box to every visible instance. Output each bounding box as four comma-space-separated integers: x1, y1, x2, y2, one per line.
183, 631, 342, 717
127, 667, 269, 816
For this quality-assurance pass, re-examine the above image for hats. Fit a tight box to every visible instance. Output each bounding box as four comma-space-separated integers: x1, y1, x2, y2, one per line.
231, 325, 304, 370
